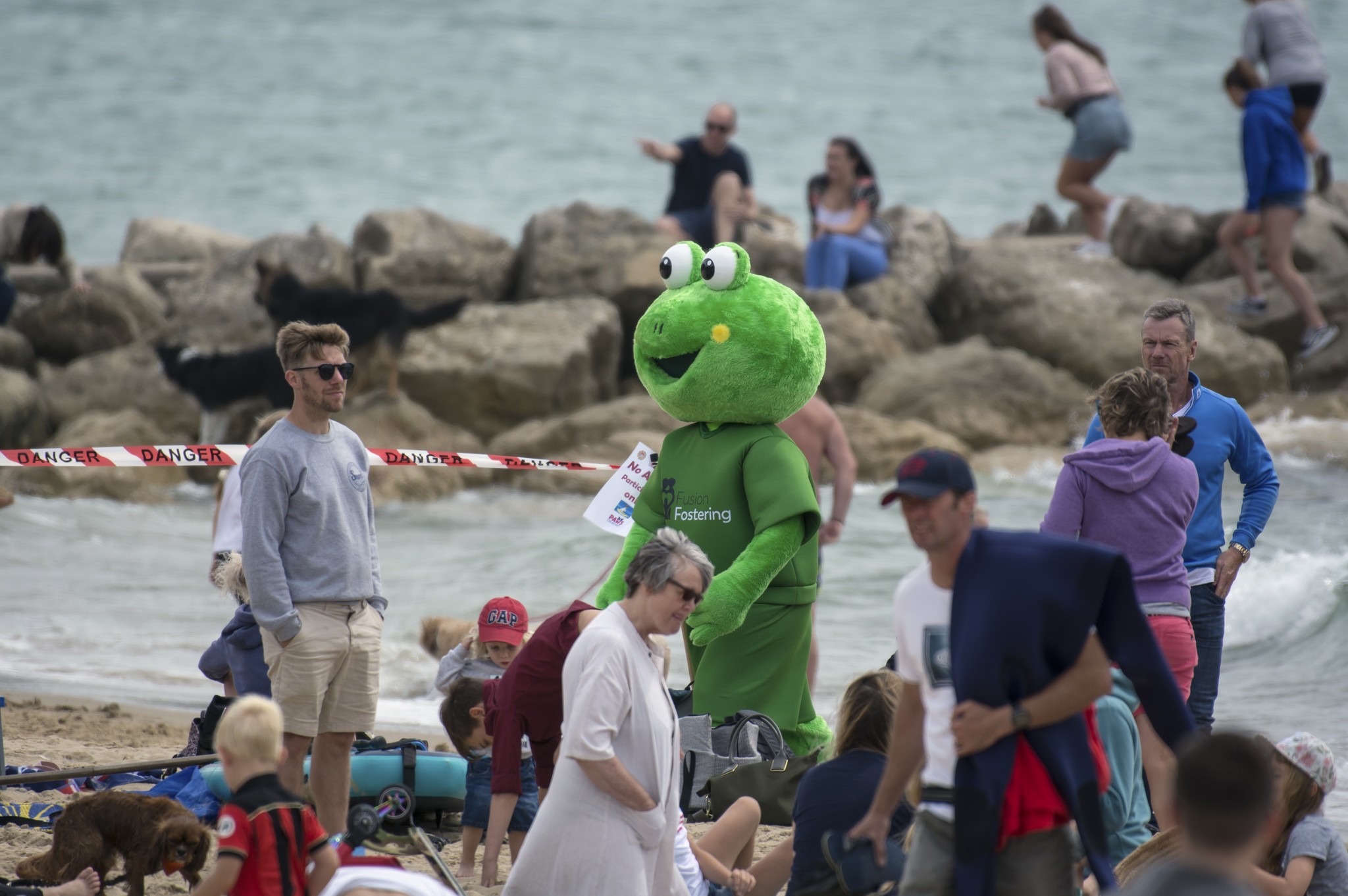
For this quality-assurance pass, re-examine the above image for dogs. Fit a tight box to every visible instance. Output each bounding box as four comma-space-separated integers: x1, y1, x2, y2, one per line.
417, 614, 486, 664
14, 786, 213, 896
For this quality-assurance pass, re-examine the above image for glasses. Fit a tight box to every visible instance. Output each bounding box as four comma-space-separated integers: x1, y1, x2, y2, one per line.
668, 576, 705, 607
705, 121, 731, 136
293, 363, 354, 382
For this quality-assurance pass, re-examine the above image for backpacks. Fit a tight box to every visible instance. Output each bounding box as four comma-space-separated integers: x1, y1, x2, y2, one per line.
724, 710, 795, 761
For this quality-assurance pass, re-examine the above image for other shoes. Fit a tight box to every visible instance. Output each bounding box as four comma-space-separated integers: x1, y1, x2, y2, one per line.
1294, 321, 1341, 358
1099, 195, 1133, 245
1224, 298, 1277, 318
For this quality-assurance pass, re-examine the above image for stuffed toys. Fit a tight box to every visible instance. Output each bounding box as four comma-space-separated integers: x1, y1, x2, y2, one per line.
592, 240, 836, 760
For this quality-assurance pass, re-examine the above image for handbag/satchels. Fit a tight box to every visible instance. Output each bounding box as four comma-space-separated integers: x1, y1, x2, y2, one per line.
696, 714, 825, 827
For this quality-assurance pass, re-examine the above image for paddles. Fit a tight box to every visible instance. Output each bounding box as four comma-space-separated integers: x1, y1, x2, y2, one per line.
0, 753, 230, 786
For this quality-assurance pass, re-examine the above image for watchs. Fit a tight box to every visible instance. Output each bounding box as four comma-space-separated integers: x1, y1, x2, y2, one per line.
1228, 542, 1251, 563
1010, 697, 1033, 731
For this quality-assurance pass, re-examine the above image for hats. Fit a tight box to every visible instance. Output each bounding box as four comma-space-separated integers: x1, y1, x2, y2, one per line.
881, 447, 975, 508
478, 596, 529, 647
1254, 731, 1338, 795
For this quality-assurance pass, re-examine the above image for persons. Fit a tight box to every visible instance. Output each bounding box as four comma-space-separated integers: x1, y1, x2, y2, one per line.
636, 104, 760, 244
239, 319, 388, 859
499, 525, 714, 896
774, 391, 854, 702
1081, 298, 1282, 735
434, 597, 542, 877
170, 694, 343, 896
1120, 730, 1284, 896
1252, 729, 1348, 896
1223, 58, 1340, 355
210, 409, 289, 604
785, 667, 916, 896
803, 136, 889, 292
1029, 4, 1134, 237
198, 550, 272, 696
845, 443, 1117, 896
1106, 663, 1176, 832
1243, 0, 1333, 191
1094, 664, 1152, 865
1039, 367, 1202, 706
0, 866, 101, 896
439, 598, 604, 887
673, 795, 793, 896
